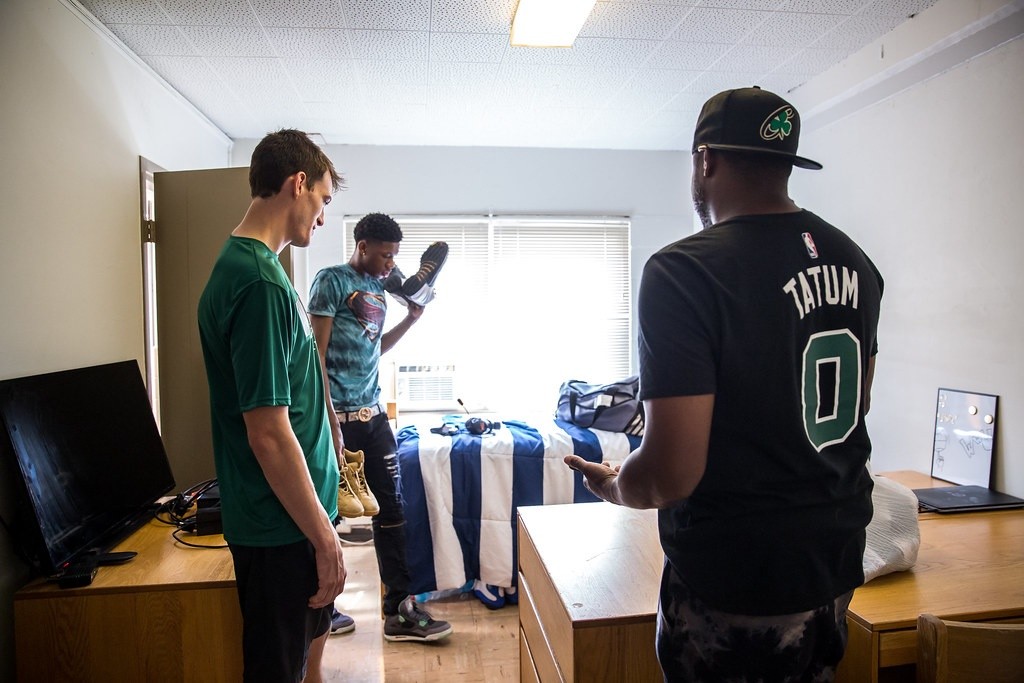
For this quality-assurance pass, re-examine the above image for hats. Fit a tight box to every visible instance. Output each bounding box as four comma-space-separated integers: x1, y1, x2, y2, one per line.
691, 85, 824, 170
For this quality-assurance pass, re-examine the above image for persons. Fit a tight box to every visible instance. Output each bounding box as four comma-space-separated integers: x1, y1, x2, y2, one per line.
192, 127, 346, 682
561, 85, 887, 683
305, 212, 453, 643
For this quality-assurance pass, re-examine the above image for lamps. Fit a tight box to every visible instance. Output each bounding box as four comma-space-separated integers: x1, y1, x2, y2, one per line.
509, 0, 598, 49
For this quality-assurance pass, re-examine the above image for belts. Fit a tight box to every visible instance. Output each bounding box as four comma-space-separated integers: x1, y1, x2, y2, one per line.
335, 404, 384, 424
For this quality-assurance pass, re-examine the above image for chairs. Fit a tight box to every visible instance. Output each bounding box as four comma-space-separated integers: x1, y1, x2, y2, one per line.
916, 613, 1024, 683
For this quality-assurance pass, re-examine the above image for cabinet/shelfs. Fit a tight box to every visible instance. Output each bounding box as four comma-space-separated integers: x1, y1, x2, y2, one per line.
516, 501, 664, 683
0, 496, 244, 683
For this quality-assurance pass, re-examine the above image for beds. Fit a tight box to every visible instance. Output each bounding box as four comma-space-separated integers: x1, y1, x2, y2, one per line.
393, 411, 642, 598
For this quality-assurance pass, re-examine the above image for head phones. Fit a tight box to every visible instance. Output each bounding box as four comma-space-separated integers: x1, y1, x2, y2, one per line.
431, 418, 501, 436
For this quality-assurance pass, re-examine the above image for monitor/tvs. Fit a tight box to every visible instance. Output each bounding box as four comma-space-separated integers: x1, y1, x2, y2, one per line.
0, 360, 177, 581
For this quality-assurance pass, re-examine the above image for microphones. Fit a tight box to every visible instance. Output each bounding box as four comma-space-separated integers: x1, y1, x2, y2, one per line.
457, 399, 470, 415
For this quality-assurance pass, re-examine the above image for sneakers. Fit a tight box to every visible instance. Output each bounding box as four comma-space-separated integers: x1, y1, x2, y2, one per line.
343, 448, 380, 516
383, 265, 411, 306
382, 604, 452, 640
328, 608, 356, 633
402, 241, 448, 305
335, 464, 365, 518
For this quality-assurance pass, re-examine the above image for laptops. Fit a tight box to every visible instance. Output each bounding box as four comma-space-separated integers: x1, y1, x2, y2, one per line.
911, 485, 1024, 514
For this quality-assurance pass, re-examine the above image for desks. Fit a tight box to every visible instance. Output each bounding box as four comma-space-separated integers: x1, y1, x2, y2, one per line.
837, 470, 1024, 683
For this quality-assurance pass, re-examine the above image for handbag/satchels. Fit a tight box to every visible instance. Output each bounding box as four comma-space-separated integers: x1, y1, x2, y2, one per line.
554, 373, 646, 439
857, 475, 922, 580
